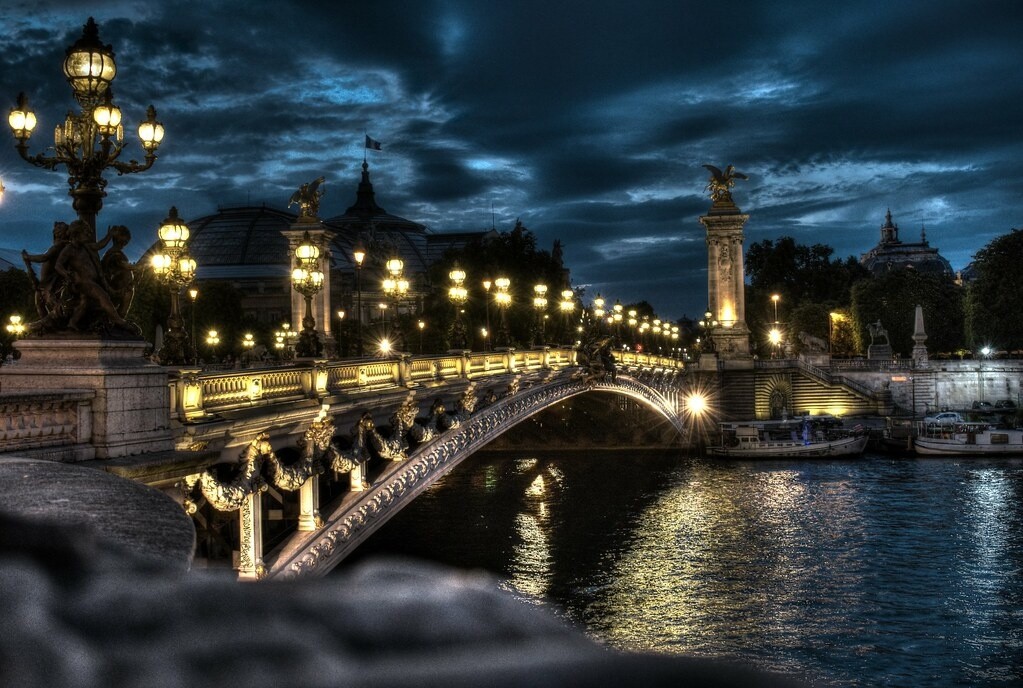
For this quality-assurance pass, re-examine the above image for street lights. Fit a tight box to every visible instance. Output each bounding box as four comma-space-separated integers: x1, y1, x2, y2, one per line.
186, 285, 200, 353
2, 11, 166, 342
772, 295, 779, 322
494, 275, 514, 349
558, 289, 574, 342
381, 256, 409, 342
613, 312, 624, 365
417, 319, 426, 356
244, 331, 256, 364
148, 205, 198, 361
445, 256, 475, 354
594, 290, 679, 348
698, 310, 718, 351
291, 229, 325, 361
338, 311, 347, 356
282, 320, 291, 362
828, 311, 837, 355
203, 326, 221, 363
379, 302, 388, 339
482, 271, 494, 350
354, 240, 366, 357
533, 281, 548, 341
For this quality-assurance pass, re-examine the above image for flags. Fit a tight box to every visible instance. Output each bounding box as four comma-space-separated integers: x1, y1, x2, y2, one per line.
801, 421, 812, 445
365, 135, 382, 150
849, 423, 863, 433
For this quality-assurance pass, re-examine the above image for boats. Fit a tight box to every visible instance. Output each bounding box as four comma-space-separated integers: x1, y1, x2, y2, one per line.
705, 414, 870, 458
915, 403, 1023, 457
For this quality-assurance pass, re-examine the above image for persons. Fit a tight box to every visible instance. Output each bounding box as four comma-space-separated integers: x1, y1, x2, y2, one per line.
22, 220, 143, 338
873, 319, 883, 337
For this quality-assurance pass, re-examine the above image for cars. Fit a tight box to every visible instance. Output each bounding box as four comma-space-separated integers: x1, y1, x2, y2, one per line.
923, 411, 964, 427
